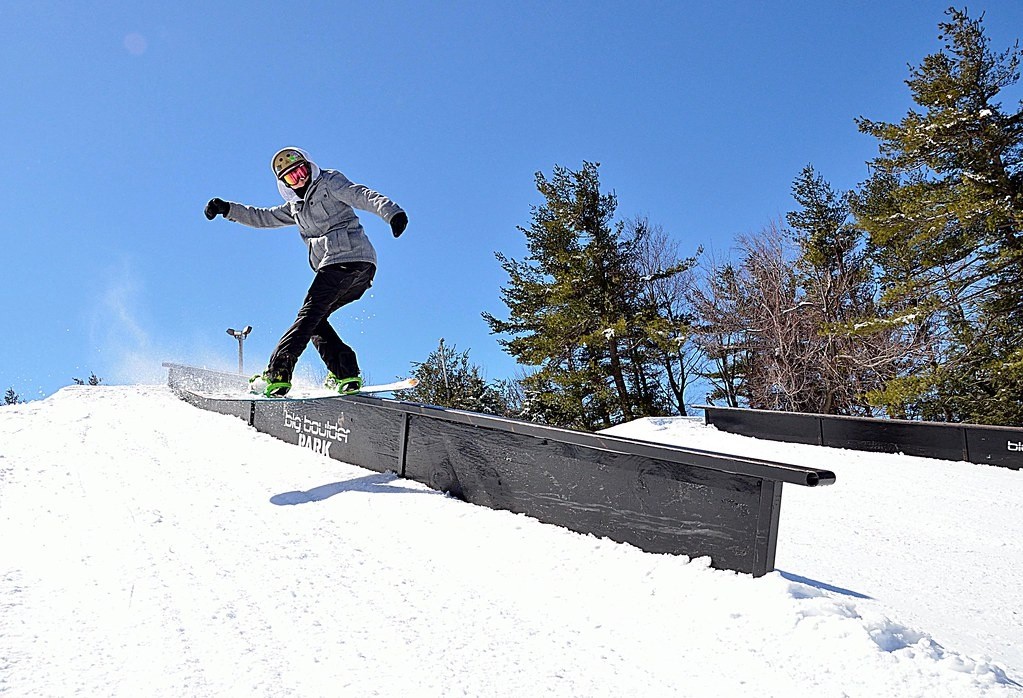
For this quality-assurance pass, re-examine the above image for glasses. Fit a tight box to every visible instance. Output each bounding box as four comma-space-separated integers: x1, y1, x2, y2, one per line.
284, 162, 310, 186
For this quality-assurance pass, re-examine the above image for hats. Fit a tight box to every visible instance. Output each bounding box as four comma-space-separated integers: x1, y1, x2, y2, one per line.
273, 150, 306, 180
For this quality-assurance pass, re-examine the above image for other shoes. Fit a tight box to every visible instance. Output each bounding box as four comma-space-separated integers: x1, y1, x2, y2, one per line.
248, 375, 289, 393
324, 374, 359, 392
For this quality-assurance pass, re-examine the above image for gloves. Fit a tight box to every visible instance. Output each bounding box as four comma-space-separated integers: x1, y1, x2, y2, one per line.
389, 212, 408, 238
204, 198, 230, 221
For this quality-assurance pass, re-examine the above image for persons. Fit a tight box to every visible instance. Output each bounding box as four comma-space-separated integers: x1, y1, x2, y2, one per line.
204, 147, 408, 400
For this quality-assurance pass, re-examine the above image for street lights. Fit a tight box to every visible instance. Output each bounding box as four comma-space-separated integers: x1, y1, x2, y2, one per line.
225, 325, 253, 376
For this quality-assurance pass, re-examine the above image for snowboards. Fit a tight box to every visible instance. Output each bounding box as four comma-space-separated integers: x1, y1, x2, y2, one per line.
184, 377, 420, 401
